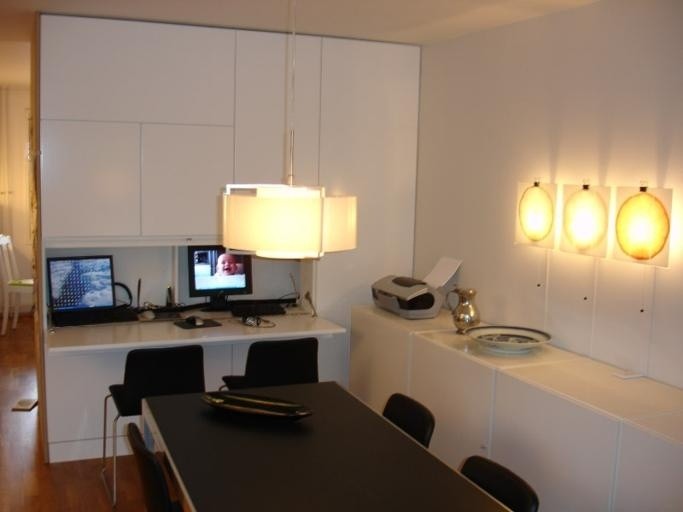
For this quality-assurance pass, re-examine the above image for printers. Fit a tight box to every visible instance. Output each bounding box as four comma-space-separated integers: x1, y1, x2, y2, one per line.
371, 275, 443, 319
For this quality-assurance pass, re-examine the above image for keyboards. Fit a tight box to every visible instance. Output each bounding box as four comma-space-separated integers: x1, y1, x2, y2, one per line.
230, 301, 285, 317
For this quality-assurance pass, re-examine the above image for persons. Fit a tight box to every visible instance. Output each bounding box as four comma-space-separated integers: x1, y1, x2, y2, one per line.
207, 253, 244, 284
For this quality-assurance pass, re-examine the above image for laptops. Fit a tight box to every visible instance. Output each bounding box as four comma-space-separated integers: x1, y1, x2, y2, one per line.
47, 255, 138, 326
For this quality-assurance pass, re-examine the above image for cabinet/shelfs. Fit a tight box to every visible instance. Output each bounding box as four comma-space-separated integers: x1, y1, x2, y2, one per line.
495, 372, 619, 510
616, 418, 682, 512
235, 26, 322, 235
41, 120, 233, 239
39, 13, 234, 125
350, 303, 412, 438
302, 35, 416, 386
410, 332, 493, 474
0, 89, 34, 236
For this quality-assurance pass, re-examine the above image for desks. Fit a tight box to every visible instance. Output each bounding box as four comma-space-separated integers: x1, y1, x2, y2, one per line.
42, 302, 349, 467
132, 381, 515, 512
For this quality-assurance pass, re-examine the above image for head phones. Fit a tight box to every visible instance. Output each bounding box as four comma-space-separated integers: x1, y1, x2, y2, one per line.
242, 312, 276, 328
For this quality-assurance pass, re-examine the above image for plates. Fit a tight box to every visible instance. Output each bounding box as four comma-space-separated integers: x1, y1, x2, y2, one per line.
461, 324, 552, 355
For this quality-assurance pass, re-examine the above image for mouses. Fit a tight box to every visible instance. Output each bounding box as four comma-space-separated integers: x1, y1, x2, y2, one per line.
142, 311, 155, 320
186, 316, 204, 326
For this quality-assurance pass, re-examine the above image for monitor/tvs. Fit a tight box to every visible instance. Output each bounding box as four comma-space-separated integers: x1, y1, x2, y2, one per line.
188, 245, 252, 311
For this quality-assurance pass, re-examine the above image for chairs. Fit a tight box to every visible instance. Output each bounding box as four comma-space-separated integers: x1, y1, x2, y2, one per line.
102, 343, 206, 508
125, 421, 187, 510
381, 391, 437, 455
457, 454, 541, 512
1, 234, 40, 332
219, 337, 320, 393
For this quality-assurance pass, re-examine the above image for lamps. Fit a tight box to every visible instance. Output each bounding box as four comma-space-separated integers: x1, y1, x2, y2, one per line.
219, 2, 359, 264
509, 177, 674, 316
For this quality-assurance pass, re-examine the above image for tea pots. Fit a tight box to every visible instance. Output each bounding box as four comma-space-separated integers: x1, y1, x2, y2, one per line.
445, 287, 481, 335
